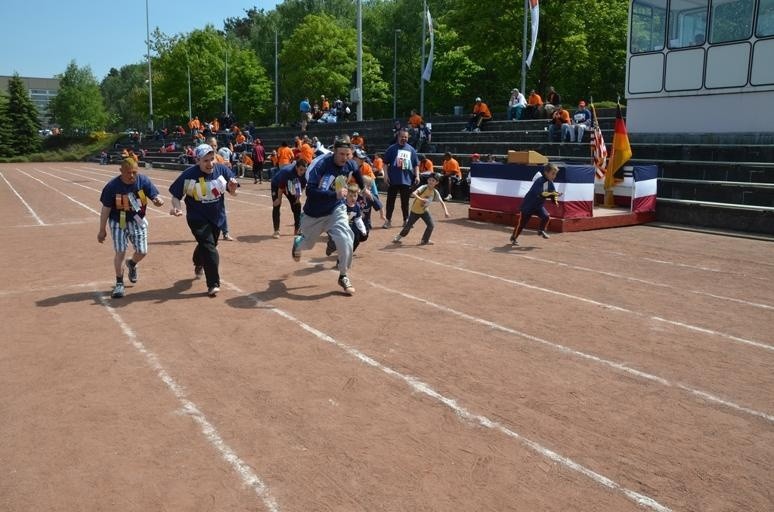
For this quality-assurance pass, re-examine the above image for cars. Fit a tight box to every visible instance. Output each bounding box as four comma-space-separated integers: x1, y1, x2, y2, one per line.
125, 127, 138, 132
37, 127, 78, 137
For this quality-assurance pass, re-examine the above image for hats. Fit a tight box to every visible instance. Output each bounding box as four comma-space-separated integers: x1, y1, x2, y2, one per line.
555, 105, 563, 111
475, 96, 482, 102
194, 143, 215, 159
579, 100, 586, 107
353, 131, 360, 137
320, 94, 325, 98
333, 138, 352, 149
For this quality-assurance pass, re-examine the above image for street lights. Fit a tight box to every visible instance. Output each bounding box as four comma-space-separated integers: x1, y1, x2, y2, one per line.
144, 79, 154, 133
392, 28, 402, 118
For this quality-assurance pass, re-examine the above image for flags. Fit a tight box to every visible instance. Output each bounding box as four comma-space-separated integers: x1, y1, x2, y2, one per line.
604, 103, 633, 190
589, 102, 608, 179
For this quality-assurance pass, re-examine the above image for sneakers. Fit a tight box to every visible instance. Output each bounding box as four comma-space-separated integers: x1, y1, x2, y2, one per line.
461, 128, 481, 132
271, 229, 304, 263
443, 194, 453, 200
538, 230, 550, 239
382, 218, 414, 229
421, 239, 435, 245
338, 276, 356, 294
195, 266, 204, 279
208, 286, 221, 296
510, 237, 520, 246
111, 282, 125, 299
125, 259, 137, 283
224, 233, 233, 241
392, 234, 402, 243
325, 246, 340, 270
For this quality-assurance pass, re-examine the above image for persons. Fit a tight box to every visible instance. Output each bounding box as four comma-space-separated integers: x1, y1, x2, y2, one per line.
279, 94, 351, 130
270, 109, 462, 267
463, 154, 496, 203
510, 164, 563, 246
291, 140, 374, 294
168, 144, 240, 295
93, 155, 167, 299
114, 115, 265, 183
463, 87, 590, 142
42, 126, 61, 136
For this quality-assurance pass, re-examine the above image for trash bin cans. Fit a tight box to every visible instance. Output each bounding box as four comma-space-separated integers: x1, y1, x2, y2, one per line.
454, 106, 464, 115
